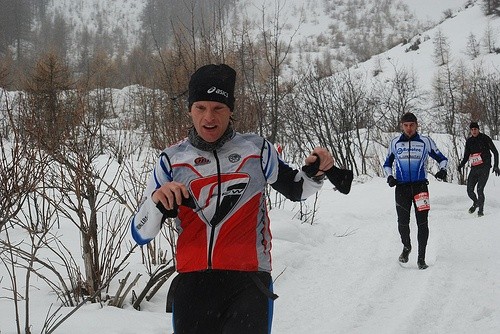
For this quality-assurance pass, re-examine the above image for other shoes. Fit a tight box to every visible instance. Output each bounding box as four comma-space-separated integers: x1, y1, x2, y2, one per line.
416, 257, 429, 270
478, 206, 484, 217
399, 248, 412, 263
468, 203, 479, 214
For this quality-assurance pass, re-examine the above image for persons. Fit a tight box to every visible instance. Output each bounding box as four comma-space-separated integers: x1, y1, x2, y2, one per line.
135, 64, 334, 334
385, 112, 448, 268
457, 122, 500, 217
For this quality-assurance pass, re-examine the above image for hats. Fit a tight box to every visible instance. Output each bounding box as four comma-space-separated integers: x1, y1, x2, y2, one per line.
469, 122, 480, 129
188, 63, 237, 112
399, 112, 418, 123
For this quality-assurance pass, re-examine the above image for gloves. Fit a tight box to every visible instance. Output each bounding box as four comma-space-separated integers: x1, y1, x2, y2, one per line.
434, 169, 447, 179
458, 164, 465, 173
491, 167, 500, 177
386, 175, 398, 187
